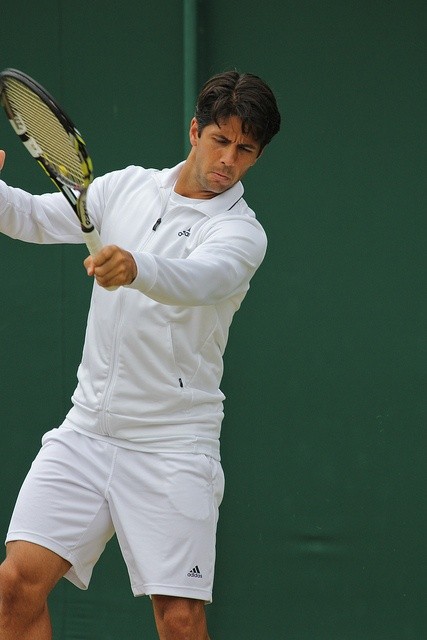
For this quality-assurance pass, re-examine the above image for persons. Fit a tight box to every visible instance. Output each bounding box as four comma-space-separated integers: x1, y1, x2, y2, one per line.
0, 70, 281, 640
